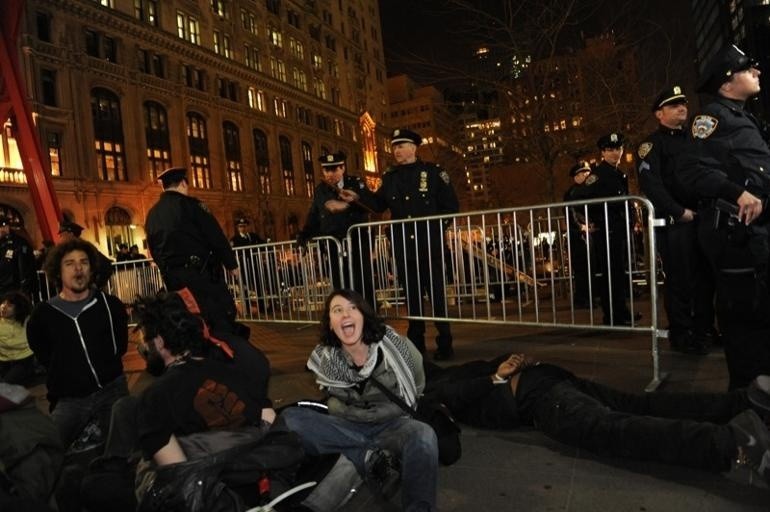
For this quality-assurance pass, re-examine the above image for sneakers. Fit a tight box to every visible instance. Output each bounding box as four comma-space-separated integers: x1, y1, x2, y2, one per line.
369, 448, 406, 502
70, 421, 105, 452
746, 375, 770, 408
727, 409, 770, 485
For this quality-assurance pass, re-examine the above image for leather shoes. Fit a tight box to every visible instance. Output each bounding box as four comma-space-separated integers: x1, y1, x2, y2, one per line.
434, 345, 454, 361
670, 323, 724, 353
603, 311, 643, 327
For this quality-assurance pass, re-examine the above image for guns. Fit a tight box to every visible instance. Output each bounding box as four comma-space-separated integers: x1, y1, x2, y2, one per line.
713, 199, 768, 231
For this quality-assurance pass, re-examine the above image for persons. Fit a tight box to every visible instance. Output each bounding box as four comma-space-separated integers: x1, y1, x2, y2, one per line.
421, 352, 769, 489
336, 126, 464, 360
567, 132, 643, 329
295, 150, 380, 332
635, 84, 724, 356
562, 162, 599, 310
661, 43, 770, 393
145, 166, 251, 341
1, 214, 439, 511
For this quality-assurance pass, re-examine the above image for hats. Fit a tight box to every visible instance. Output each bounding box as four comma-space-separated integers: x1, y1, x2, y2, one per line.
595, 132, 625, 150
651, 86, 689, 112
57, 220, 84, 235
157, 168, 189, 186
236, 219, 249, 226
694, 39, 759, 95
391, 128, 422, 147
0, 217, 12, 228
569, 160, 592, 177
320, 154, 347, 169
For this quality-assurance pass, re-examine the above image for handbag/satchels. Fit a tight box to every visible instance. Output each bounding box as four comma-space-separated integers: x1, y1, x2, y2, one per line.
414, 397, 462, 466
